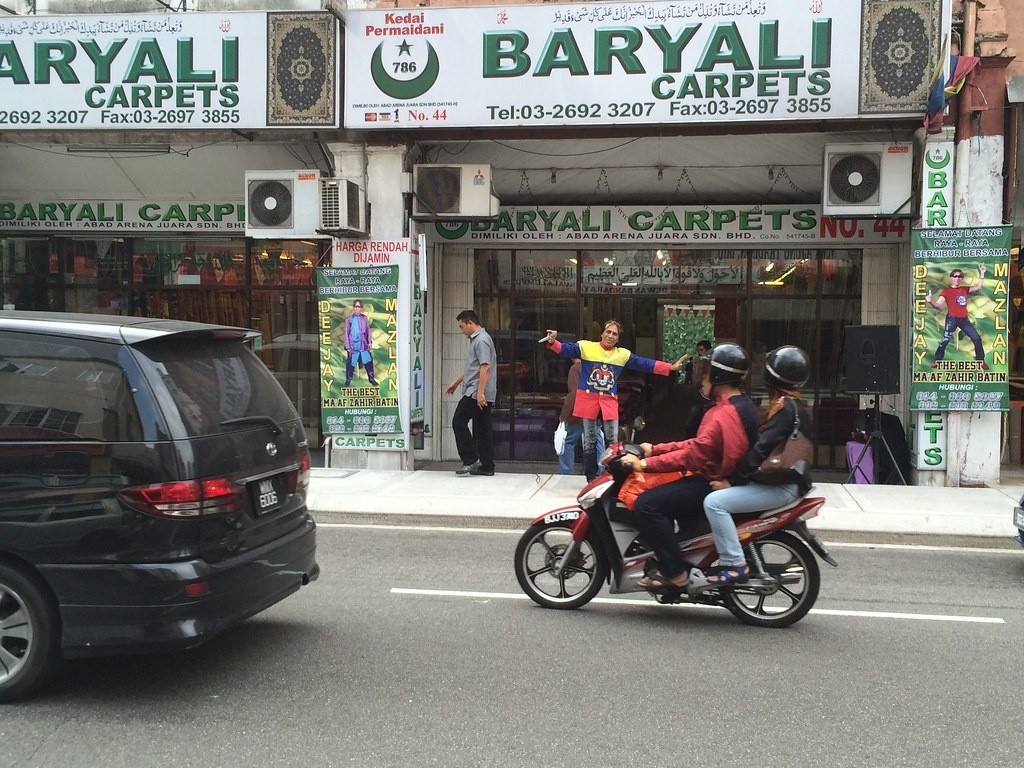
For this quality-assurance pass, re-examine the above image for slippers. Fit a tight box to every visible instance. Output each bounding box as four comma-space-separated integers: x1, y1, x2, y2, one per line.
636, 570, 692, 590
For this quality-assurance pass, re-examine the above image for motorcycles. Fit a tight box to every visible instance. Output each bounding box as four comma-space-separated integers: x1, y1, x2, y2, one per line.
511, 415, 841, 629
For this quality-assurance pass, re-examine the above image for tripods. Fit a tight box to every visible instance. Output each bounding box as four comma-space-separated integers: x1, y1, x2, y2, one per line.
845, 394, 907, 486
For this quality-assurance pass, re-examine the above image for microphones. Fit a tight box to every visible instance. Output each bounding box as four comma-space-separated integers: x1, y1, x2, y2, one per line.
538, 333, 556, 343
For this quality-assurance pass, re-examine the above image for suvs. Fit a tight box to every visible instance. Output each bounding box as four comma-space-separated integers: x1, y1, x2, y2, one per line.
0, 310, 321, 702
252, 339, 320, 419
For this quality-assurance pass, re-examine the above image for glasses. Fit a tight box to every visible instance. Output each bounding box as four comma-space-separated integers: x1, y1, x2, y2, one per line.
952, 275, 963, 278
353, 304, 363, 309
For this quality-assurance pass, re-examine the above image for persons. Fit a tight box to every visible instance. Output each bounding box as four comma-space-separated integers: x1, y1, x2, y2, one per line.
924, 263, 992, 372
620, 343, 759, 589
9, 272, 50, 310
616, 384, 635, 424
676, 338, 716, 436
444, 309, 497, 476
544, 319, 688, 485
343, 300, 378, 385
704, 342, 815, 580
556, 355, 606, 476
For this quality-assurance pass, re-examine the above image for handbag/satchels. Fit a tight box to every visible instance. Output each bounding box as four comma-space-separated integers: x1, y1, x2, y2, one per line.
752, 395, 814, 486
553, 420, 568, 456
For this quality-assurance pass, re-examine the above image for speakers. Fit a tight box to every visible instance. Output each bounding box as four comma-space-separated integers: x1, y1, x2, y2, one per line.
843, 324, 900, 394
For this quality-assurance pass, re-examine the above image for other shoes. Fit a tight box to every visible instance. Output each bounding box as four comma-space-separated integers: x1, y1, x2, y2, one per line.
345, 381, 350, 386
932, 361, 938, 367
371, 380, 378, 386
981, 363, 989, 370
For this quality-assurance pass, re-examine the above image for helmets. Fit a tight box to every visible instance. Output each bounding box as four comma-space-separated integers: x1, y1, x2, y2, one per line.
700, 341, 752, 386
764, 345, 813, 391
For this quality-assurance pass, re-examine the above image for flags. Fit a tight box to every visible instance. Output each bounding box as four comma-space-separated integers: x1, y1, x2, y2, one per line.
924, 39, 979, 135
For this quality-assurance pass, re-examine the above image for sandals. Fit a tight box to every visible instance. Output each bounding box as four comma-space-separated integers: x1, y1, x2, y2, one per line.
706, 563, 754, 584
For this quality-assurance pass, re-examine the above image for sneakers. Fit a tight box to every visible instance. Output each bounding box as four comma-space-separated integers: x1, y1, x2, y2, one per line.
469, 468, 494, 476
456, 458, 483, 474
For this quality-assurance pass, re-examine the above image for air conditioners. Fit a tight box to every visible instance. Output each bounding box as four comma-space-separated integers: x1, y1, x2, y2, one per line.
822, 142, 914, 217
244, 169, 327, 239
413, 162, 492, 218
316, 177, 365, 238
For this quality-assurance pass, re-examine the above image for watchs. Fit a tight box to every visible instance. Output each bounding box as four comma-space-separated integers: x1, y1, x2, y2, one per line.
640, 458, 649, 474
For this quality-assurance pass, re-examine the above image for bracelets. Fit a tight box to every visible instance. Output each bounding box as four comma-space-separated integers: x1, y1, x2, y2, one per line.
979, 276, 985, 280
